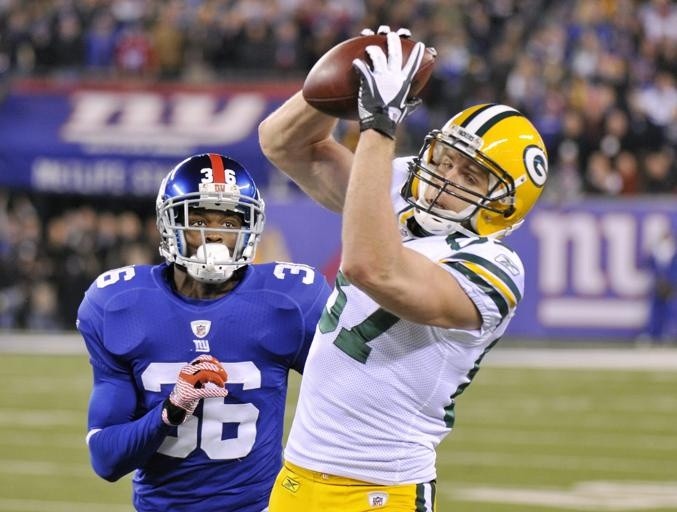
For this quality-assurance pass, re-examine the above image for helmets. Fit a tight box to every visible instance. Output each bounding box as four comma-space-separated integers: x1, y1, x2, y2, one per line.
155, 151, 266, 285
413, 103, 550, 237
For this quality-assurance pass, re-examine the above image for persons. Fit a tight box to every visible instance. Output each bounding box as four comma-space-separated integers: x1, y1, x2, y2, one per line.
73, 151, 331, 512
252, 24, 549, 510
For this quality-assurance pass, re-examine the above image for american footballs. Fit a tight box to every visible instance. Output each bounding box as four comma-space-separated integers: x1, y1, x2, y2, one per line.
303, 34, 436, 121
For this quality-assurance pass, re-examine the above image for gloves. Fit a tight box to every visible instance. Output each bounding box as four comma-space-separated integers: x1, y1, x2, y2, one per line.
351, 24, 423, 139
161, 354, 229, 427
